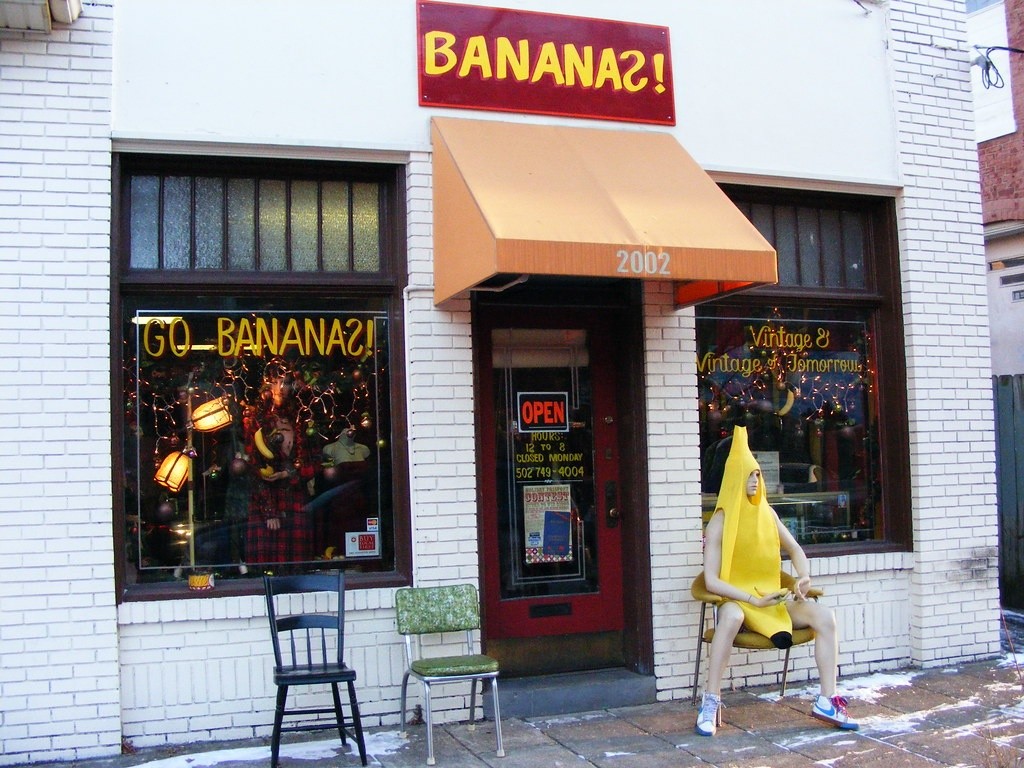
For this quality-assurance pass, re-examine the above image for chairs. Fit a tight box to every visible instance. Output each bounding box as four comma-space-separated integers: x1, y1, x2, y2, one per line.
396, 584, 505, 765
691, 569, 824, 704
264, 573, 368, 768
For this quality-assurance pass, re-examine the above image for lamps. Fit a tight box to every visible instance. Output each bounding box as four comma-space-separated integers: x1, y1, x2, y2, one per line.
192, 398, 233, 433
154, 451, 190, 493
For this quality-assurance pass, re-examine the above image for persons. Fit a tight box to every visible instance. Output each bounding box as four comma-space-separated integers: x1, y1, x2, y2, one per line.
222, 439, 252, 566
242, 360, 318, 563
324, 429, 374, 558
697, 424, 859, 735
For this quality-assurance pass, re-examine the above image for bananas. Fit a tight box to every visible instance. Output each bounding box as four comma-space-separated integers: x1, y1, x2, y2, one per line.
254, 427, 273, 458
326, 547, 335, 559
778, 389, 794, 415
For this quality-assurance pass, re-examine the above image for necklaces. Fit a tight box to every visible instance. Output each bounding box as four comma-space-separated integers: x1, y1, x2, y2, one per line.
338, 440, 358, 457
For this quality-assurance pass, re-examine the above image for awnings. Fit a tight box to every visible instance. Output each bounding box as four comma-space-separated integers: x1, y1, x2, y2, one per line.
431, 119, 780, 306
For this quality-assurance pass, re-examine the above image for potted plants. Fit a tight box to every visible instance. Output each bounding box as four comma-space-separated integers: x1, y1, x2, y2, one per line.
188, 567, 217, 591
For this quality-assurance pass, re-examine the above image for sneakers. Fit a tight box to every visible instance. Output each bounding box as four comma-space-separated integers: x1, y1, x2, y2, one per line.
695, 692, 726, 736
812, 695, 860, 731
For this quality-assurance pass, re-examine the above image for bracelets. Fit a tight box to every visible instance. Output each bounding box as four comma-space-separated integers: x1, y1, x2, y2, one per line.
748, 595, 753, 602
